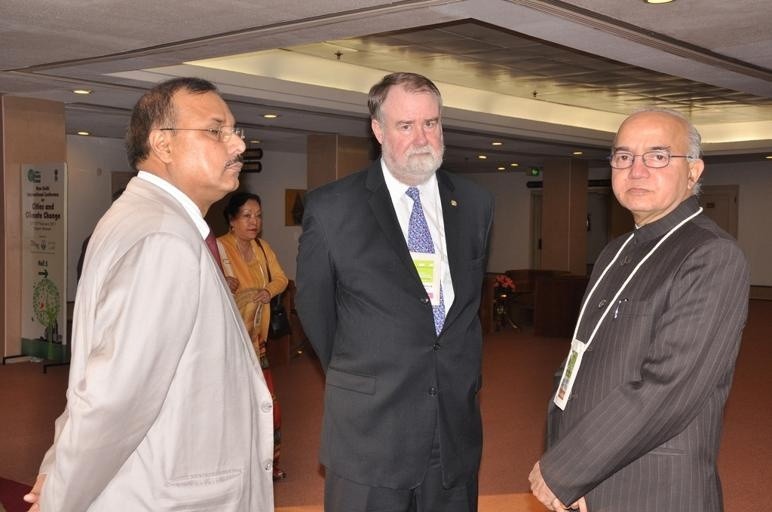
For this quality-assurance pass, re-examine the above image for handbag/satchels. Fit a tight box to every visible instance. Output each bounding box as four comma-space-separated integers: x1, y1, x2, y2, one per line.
268, 294, 289, 339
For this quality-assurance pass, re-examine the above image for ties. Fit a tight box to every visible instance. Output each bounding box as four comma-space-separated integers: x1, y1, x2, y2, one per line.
406, 186, 445, 336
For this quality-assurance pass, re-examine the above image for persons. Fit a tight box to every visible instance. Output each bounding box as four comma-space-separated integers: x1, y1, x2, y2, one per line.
22, 75, 278, 512
296, 70, 496, 512
215, 190, 290, 484
524, 103, 753, 512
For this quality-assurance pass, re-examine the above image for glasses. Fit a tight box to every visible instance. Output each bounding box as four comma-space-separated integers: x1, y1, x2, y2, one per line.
157, 126, 247, 143
606, 147, 694, 170
